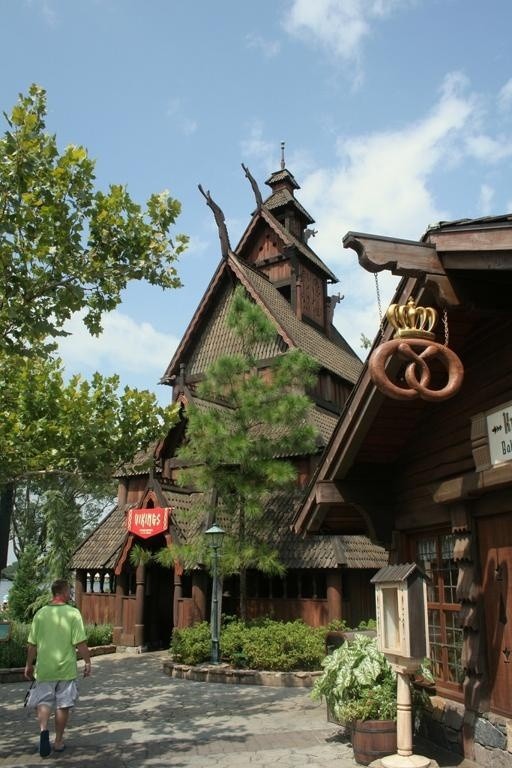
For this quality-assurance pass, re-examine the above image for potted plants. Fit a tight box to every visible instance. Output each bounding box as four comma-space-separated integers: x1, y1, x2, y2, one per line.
349, 663, 418, 766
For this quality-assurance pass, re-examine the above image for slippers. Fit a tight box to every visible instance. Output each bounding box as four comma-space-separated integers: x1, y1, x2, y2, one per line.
39, 730, 51, 758
52, 741, 66, 754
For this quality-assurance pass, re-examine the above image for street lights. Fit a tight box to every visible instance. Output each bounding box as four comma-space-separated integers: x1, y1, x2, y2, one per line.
205, 523, 228, 668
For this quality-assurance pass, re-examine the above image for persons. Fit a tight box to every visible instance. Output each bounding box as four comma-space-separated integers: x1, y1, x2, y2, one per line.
21, 579, 94, 757
